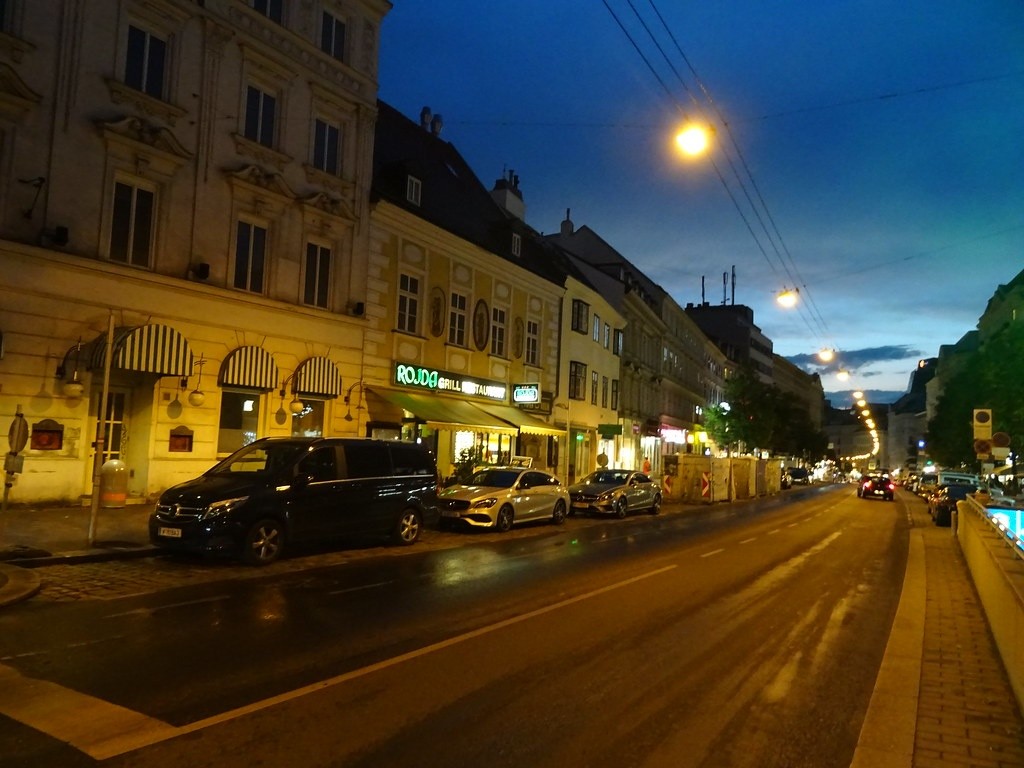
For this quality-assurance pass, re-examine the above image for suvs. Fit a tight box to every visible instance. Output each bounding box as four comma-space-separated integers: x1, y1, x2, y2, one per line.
787, 467, 808, 486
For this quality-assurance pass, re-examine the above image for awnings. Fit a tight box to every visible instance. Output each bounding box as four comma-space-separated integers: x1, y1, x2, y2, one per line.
366, 382, 568, 436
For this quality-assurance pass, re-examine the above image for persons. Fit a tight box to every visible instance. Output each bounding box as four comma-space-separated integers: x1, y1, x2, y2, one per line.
642, 456, 651, 473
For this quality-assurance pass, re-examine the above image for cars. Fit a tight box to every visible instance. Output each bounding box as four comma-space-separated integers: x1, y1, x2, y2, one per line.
438, 467, 571, 532
568, 470, 664, 519
893, 472, 1016, 527
858, 476, 894, 501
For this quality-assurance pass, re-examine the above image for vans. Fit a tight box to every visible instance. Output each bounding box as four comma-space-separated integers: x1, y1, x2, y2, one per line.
149, 437, 440, 566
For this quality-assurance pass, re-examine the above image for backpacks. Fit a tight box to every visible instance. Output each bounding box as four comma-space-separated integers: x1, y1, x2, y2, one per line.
645, 462, 651, 472
